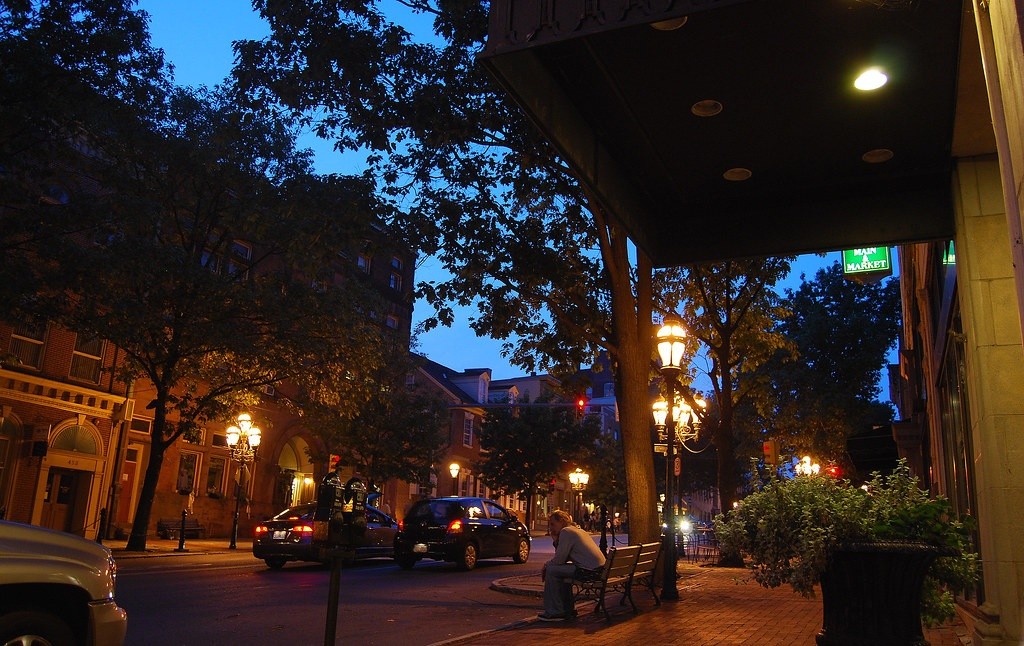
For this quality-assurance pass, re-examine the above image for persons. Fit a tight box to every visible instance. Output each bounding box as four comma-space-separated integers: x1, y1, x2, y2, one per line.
537, 510, 606, 622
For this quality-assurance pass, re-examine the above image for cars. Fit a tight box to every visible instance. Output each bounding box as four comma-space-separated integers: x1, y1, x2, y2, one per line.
0, 519, 127, 646
252, 500, 399, 568
662, 512, 703, 535
394, 496, 531, 572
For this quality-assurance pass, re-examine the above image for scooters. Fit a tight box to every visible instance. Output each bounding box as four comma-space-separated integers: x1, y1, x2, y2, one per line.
604, 511, 612, 534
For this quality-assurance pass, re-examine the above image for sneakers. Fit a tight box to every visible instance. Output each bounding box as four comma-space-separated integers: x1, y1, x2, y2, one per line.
538, 611, 565, 620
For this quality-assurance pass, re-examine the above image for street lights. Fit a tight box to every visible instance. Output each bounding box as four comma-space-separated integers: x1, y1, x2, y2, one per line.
569, 468, 590, 525
450, 463, 460, 495
656, 310, 689, 600
225, 412, 261, 549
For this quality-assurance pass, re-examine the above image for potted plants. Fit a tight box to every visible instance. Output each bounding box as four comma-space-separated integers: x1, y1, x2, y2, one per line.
713, 454, 982, 646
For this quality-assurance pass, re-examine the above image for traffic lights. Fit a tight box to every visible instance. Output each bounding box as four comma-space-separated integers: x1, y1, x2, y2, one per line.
549, 478, 556, 492
577, 397, 586, 420
761, 440, 775, 469
329, 454, 341, 475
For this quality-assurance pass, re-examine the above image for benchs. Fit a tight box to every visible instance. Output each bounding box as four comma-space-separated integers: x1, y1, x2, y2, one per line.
571, 539, 664, 627
159, 518, 205, 540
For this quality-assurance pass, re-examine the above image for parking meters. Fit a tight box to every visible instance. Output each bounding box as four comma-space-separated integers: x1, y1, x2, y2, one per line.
317, 474, 369, 646
